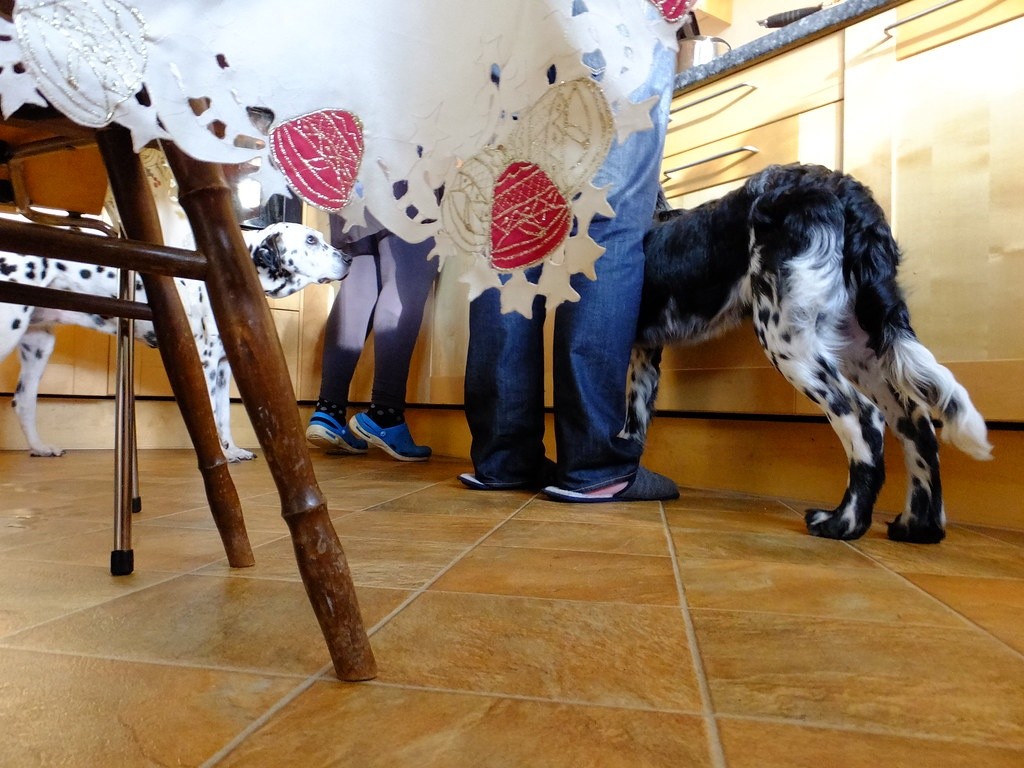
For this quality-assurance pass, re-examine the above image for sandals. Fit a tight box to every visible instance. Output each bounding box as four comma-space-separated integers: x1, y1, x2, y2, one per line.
305, 412, 370, 452
349, 412, 432, 461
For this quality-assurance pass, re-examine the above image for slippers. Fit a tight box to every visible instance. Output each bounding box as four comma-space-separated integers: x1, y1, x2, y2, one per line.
458, 457, 679, 502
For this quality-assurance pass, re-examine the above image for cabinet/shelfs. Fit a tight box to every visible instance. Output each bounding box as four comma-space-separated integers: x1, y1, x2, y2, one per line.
0, 205, 109, 397
107, 288, 304, 398
299, 204, 554, 409
843, 0, 1024, 422
653, 29, 843, 416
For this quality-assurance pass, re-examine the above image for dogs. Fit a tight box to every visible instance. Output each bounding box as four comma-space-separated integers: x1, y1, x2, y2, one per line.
626, 158, 994, 543
0, 205, 352, 463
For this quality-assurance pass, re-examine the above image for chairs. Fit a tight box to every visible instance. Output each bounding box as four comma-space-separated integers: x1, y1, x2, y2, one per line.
0, 125, 142, 578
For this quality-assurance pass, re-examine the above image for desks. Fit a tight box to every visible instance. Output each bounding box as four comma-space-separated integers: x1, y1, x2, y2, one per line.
0, 0, 695, 682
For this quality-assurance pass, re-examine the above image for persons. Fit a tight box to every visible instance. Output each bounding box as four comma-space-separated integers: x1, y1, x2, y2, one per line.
456, 0, 690, 503
303, 146, 446, 462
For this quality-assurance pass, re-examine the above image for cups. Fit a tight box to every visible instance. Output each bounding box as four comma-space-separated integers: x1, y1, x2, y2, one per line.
676, 35, 731, 72
